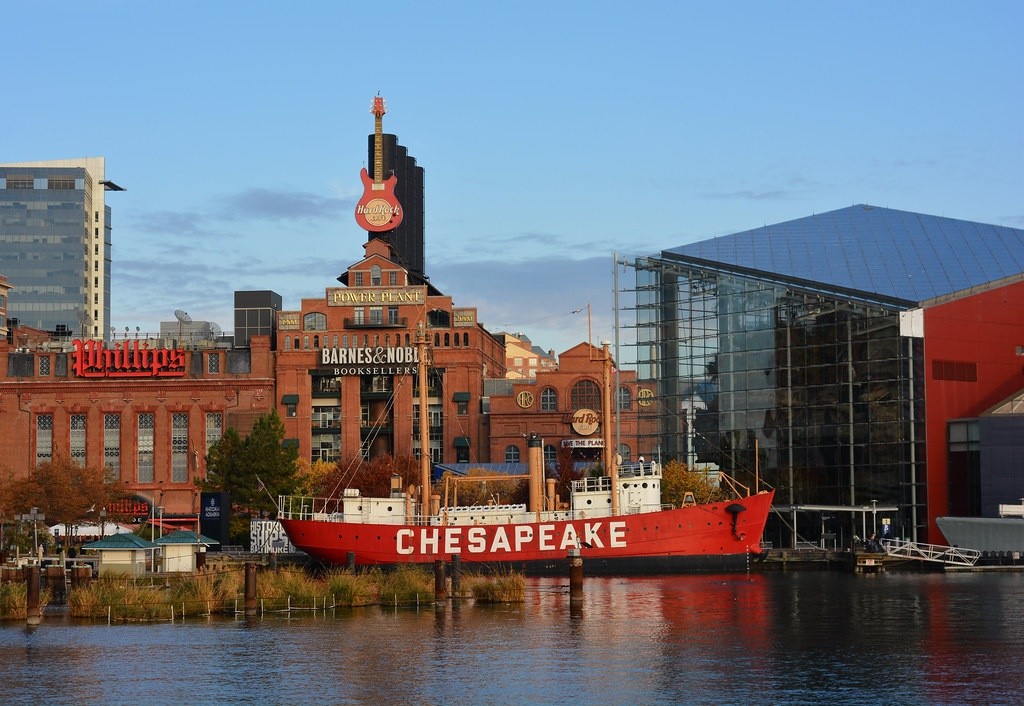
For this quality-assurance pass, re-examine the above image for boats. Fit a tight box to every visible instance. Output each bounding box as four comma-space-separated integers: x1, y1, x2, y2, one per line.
276, 337, 776, 573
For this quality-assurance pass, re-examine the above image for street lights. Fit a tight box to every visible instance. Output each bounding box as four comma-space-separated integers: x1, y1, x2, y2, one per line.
75, 523, 78, 536
791, 504, 802, 550
870, 500, 879, 534
99, 510, 107, 540
32, 507, 39, 550
157, 506, 165, 538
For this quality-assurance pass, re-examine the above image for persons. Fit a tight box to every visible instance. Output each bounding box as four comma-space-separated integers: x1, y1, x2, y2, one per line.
66, 545, 77, 558
